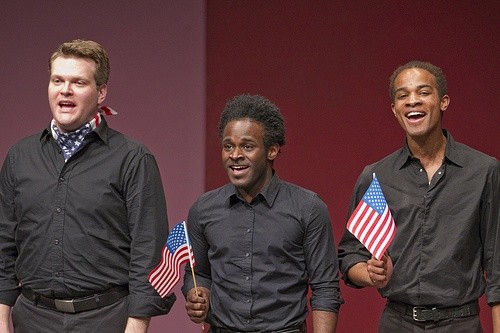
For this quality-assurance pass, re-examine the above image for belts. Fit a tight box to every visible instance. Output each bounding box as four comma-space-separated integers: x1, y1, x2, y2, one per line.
21, 287, 129, 314
386, 300, 478, 323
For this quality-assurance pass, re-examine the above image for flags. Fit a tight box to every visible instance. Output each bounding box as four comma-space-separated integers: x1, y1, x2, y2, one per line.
345, 175, 396, 261
146, 218, 196, 298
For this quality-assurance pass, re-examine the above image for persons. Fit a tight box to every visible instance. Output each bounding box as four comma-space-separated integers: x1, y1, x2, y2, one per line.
338, 61, 500, 333
1, 38, 170, 333
180, 96, 344, 333
